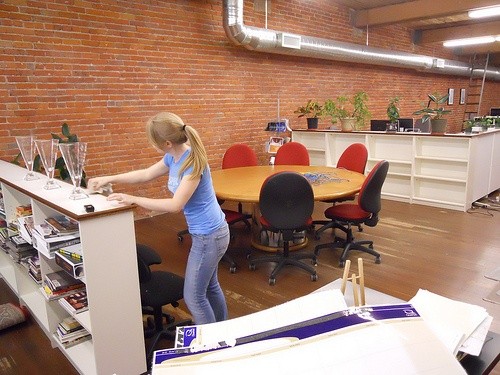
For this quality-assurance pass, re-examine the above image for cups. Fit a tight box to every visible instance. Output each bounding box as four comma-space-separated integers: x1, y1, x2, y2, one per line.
266, 119, 291, 132
15, 135, 41, 181
33, 138, 62, 190
58, 142, 90, 200
266, 137, 290, 153
269, 156, 276, 165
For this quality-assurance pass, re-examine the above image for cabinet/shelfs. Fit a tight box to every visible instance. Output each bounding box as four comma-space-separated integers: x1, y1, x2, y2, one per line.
291, 127, 500, 211
0, 159, 147, 375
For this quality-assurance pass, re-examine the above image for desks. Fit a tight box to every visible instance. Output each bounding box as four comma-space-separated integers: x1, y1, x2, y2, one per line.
211, 165, 366, 251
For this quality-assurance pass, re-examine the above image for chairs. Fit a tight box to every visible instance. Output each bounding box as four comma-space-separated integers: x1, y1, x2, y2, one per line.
178, 143, 389, 284
136, 244, 191, 367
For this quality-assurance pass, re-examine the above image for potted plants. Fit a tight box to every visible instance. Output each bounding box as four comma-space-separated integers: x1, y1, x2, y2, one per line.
384, 99, 398, 134
464, 115, 487, 132
428, 99, 448, 133
333, 98, 362, 131
301, 101, 322, 128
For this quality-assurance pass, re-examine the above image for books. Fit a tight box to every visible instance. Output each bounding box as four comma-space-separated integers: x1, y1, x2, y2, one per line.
0, 187, 89, 349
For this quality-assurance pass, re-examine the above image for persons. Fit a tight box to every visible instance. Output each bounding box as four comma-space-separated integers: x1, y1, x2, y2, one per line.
88, 112, 230, 324
